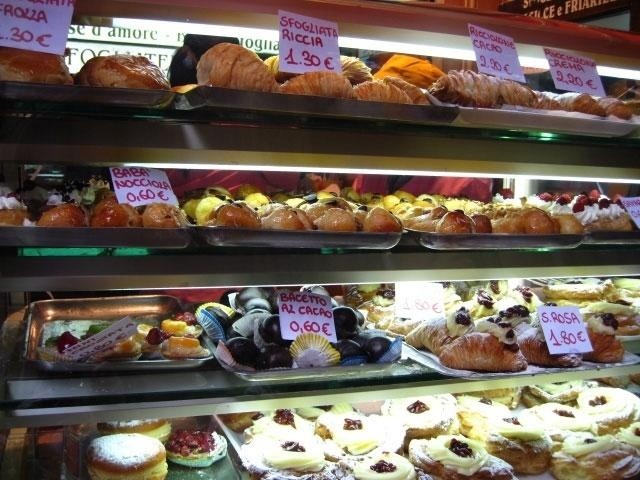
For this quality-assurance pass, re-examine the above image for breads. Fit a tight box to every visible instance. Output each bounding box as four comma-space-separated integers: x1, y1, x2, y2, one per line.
280, 70, 355, 99
206, 286, 345, 376
346, 280, 640, 373
38, 201, 87, 228
263, 55, 282, 74
89, 196, 141, 227
72, 53, 171, 92
430, 70, 633, 121
162, 428, 227, 471
402, 187, 492, 233
0, 46, 72, 83
185, 183, 402, 232
223, 381, 640, 480
195, 41, 282, 93
83, 420, 173, 480
142, 201, 186, 230
341, 49, 445, 105
1, 195, 28, 228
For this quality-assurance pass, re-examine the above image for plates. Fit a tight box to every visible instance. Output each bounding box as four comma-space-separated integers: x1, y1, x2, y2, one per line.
402, 339, 637, 383
203, 226, 403, 251
2, 79, 180, 111
180, 84, 461, 127
5, 224, 192, 249
21, 294, 214, 371
456, 105, 639, 139
205, 335, 407, 385
581, 230, 640, 247
214, 413, 270, 471
77, 430, 242, 480
405, 229, 588, 251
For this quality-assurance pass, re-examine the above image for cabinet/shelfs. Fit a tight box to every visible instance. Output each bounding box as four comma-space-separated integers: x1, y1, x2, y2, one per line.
0, 1, 639, 480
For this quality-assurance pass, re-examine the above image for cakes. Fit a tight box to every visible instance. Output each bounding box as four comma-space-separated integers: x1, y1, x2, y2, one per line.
53, 326, 141, 363
492, 187, 630, 234
164, 334, 209, 362
137, 324, 165, 357
161, 308, 202, 341
